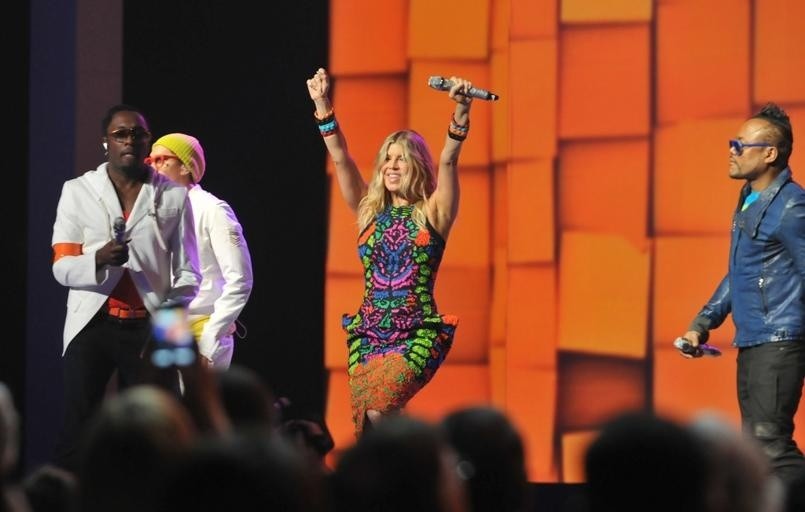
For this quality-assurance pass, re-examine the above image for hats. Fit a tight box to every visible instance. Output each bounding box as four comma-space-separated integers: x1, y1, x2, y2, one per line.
151, 133, 206, 185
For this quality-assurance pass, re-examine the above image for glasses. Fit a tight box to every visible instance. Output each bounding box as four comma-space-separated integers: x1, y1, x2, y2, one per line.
109, 129, 152, 144
143, 155, 178, 168
730, 140, 771, 156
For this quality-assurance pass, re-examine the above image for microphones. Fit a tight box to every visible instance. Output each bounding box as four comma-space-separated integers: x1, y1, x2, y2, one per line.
673, 336, 724, 359
428, 75, 500, 103
112, 216, 126, 245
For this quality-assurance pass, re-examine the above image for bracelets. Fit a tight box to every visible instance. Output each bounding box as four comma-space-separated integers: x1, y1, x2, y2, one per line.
313, 107, 339, 137
448, 113, 470, 142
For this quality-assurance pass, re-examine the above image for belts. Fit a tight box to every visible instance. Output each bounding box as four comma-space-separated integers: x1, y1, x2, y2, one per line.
108, 307, 146, 319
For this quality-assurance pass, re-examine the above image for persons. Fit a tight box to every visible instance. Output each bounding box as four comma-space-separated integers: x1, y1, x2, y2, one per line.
51, 105, 203, 431
142, 133, 254, 376
1, 364, 785, 511
307, 68, 472, 440
679, 104, 804, 512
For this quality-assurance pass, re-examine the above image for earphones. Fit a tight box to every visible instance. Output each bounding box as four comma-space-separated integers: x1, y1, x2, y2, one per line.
103, 142, 108, 150
